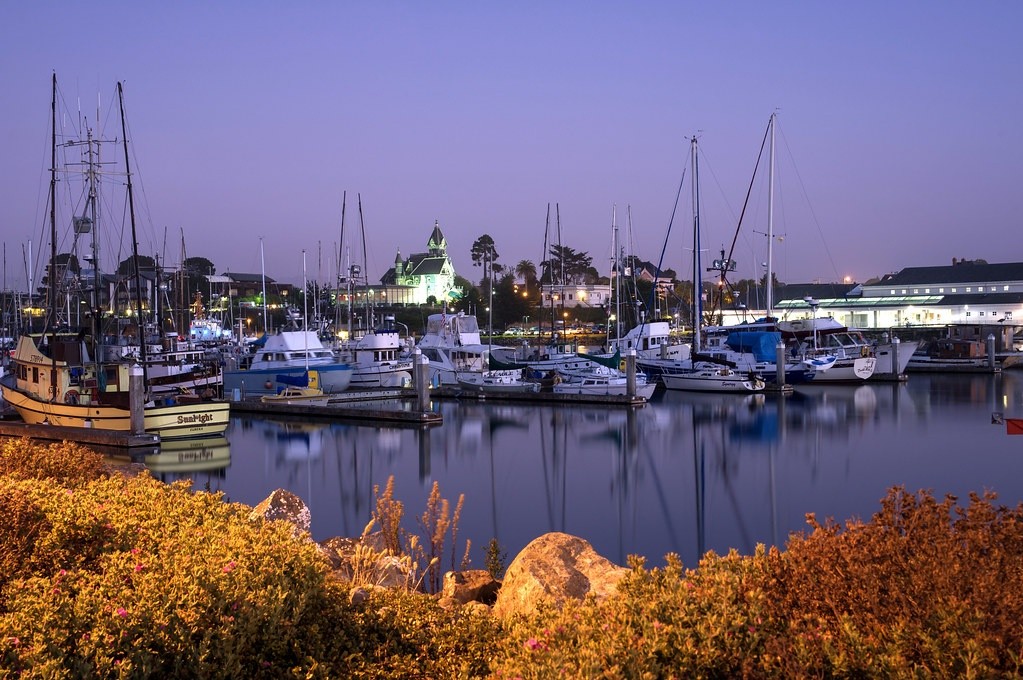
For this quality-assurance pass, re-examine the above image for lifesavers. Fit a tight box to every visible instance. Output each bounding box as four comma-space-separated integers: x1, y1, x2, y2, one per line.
861, 345, 869, 357
553, 376, 563, 385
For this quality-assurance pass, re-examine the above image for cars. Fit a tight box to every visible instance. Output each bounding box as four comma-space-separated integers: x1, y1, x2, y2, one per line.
504, 328, 516, 335
591, 324, 606, 334
482, 329, 500, 336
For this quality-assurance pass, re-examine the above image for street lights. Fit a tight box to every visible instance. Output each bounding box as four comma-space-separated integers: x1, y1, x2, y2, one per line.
964, 305, 968, 339
524, 316, 530, 335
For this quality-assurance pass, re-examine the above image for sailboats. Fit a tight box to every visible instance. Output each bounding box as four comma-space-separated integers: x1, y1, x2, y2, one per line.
0, 68, 232, 439
309, 191, 692, 389
577, 112, 928, 385
261, 248, 333, 407
223, 237, 354, 394
457, 248, 542, 393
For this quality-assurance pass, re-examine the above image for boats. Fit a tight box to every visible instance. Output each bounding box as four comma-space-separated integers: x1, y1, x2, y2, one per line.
903, 338, 1002, 374
551, 376, 658, 402
660, 216, 766, 392
122, 252, 224, 398
